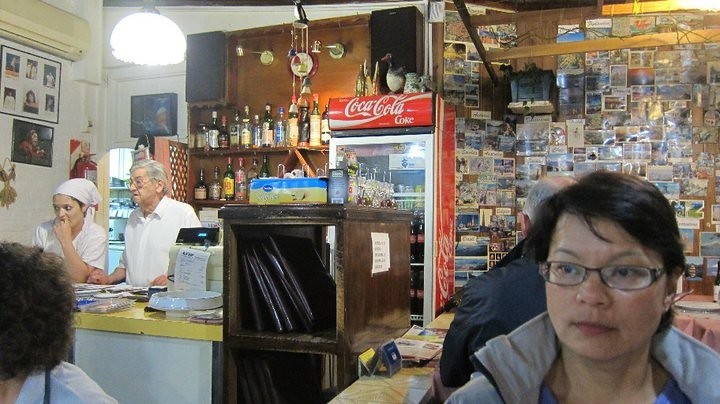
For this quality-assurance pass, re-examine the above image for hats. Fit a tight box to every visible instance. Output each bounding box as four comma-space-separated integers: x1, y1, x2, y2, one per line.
53, 178, 101, 206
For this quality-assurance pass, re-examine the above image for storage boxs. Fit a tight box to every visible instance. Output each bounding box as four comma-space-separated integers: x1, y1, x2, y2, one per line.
328, 168, 348, 205
247, 175, 328, 208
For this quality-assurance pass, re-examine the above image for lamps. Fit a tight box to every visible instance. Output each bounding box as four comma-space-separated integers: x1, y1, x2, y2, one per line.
108, 0, 187, 67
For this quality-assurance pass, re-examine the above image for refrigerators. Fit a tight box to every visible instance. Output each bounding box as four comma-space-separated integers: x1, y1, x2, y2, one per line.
327, 91, 456, 327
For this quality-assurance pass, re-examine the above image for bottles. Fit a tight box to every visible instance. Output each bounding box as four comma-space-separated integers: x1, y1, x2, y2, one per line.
320, 104, 331, 145
218, 116, 229, 148
298, 101, 310, 146
241, 105, 252, 148
223, 157, 234, 201
355, 65, 372, 97
285, 96, 299, 146
298, 78, 313, 121
274, 107, 284, 147
195, 124, 208, 148
261, 104, 274, 147
310, 94, 321, 146
247, 160, 258, 199
234, 158, 247, 201
411, 215, 425, 263
229, 109, 241, 148
208, 111, 219, 148
410, 268, 424, 314
194, 168, 208, 200
252, 115, 260, 148
208, 166, 221, 200
372, 61, 381, 95
259, 155, 269, 177
395, 185, 425, 209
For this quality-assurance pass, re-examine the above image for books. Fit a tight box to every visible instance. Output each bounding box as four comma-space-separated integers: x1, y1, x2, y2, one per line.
75, 285, 147, 313
394, 324, 448, 364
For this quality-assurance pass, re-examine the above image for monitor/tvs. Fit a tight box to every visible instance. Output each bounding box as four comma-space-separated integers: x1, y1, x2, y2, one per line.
130, 93, 177, 137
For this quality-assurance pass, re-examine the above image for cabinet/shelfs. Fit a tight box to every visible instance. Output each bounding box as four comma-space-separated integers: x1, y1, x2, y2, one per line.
186, 144, 330, 206
108, 186, 141, 251
216, 204, 416, 404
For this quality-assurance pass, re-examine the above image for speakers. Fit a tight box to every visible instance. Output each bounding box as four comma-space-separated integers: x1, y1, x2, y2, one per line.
371, 6, 424, 94
185, 30, 227, 101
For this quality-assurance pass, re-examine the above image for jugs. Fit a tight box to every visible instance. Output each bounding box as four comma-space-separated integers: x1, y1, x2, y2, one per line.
402, 73, 424, 93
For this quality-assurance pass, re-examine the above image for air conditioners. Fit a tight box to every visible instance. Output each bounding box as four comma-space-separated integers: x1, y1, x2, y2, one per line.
0, 0, 93, 63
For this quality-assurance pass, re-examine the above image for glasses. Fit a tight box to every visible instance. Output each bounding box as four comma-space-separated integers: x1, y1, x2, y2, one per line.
127, 178, 155, 188
538, 261, 673, 290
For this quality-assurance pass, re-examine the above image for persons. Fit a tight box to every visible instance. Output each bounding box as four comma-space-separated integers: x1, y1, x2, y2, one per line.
32, 178, 108, 282
47, 97, 53, 112
151, 106, 169, 133
19, 130, 45, 158
440, 175, 578, 387
24, 89, 38, 113
27, 63, 36, 78
9, 56, 16, 71
445, 170, 720, 404
87, 159, 202, 286
4, 90, 15, 106
0, 241, 116, 404
46, 69, 54, 87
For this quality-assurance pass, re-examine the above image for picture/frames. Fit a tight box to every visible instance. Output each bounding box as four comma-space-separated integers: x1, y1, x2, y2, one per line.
0, 44, 62, 125
10, 118, 54, 169
